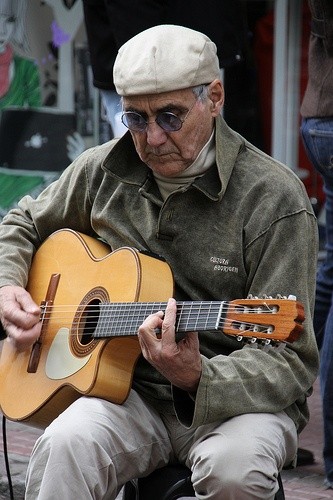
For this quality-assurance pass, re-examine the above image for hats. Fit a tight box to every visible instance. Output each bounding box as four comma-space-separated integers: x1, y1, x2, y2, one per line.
112, 24, 221, 95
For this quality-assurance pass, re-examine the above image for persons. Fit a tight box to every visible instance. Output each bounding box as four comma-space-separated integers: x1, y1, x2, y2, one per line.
0, 25, 323, 499
0, 1, 326, 222
274, 0, 332, 469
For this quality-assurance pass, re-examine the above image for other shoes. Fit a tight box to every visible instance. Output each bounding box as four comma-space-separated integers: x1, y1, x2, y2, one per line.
282, 447, 315, 470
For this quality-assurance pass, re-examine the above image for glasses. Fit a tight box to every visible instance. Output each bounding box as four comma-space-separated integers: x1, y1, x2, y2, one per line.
121, 87, 207, 132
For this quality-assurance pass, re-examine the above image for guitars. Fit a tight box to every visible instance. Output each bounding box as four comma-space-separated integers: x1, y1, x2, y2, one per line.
0, 226, 307, 431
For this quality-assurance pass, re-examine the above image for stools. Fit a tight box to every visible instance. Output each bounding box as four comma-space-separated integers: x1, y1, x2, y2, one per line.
122, 453, 287, 500
